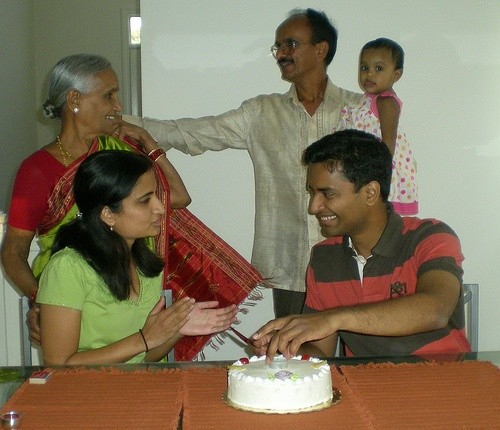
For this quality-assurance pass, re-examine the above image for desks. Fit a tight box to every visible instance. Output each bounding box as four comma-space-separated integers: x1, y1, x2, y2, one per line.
0, 350, 500, 430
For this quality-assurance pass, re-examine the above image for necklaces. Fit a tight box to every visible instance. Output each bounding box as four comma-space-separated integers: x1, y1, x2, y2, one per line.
55, 135, 72, 168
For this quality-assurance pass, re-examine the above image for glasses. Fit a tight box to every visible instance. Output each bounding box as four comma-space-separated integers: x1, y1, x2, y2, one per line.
270, 39, 316, 60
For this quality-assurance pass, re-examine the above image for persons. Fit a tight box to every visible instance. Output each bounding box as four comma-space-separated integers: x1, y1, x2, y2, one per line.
333, 38, 418, 215
252, 129, 472, 364
118, 8, 364, 356
0, 54, 192, 366
35, 150, 237, 367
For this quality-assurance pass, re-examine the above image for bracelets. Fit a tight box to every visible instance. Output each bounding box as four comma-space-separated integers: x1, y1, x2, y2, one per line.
28, 287, 38, 309
138, 328, 149, 354
147, 148, 166, 162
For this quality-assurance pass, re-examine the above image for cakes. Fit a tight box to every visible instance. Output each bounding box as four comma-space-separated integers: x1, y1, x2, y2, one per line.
227, 355, 333, 411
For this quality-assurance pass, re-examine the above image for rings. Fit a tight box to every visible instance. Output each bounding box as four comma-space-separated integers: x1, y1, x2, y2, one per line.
112, 130, 119, 135
